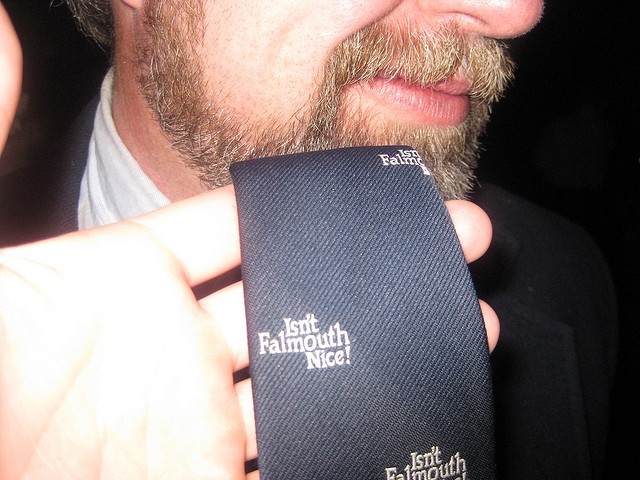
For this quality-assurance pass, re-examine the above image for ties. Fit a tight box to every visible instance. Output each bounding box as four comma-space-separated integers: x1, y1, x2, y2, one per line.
227, 145, 497, 480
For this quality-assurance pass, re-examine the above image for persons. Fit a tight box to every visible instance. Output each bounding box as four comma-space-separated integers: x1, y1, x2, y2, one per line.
0, 0, 617, 476
0, 4, 500, 480
